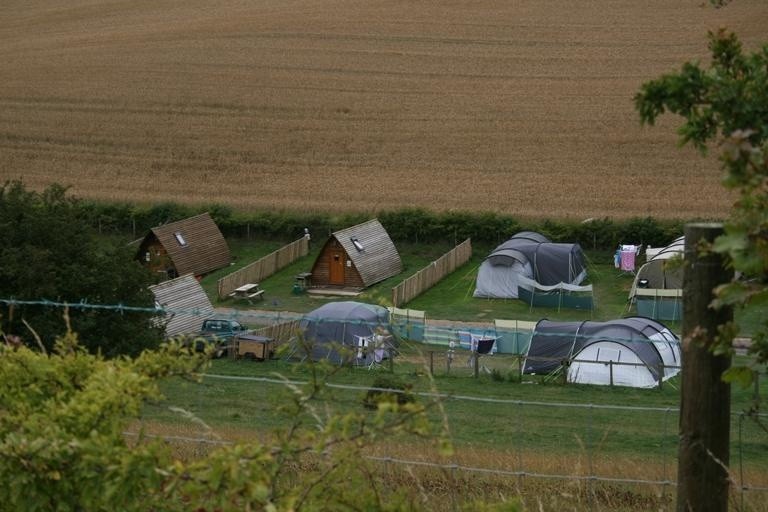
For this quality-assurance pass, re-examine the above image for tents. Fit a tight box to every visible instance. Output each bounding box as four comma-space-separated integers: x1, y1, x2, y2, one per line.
471, 230, 592, 301
627, 234, 686, 321
521, 315, 681, 390
286, 302, 400, 365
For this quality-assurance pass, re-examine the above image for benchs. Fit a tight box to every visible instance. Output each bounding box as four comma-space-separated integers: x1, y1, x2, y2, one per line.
228, 289, 264, 305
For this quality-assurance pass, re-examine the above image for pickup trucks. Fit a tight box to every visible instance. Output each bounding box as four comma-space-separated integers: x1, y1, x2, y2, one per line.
188, 317, 248, 360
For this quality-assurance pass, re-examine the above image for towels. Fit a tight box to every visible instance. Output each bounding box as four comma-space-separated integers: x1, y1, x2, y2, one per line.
458, 332, 498, 355
620, 251, 636, 272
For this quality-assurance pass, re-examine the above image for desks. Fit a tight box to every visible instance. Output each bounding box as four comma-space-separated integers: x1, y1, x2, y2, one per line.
235, 283, 264, 306
